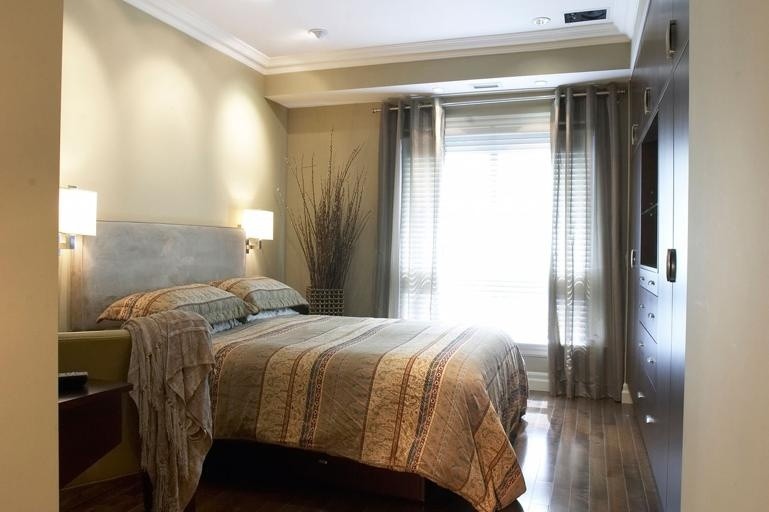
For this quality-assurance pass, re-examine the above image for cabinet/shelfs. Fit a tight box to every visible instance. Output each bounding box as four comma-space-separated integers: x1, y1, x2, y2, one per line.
628, 0, 688, 512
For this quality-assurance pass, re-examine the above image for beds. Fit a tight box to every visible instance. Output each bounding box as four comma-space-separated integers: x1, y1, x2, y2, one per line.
74, 222, 517, 512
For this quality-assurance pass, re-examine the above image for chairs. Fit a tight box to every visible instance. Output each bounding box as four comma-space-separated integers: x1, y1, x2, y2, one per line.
59, 327, 197, 512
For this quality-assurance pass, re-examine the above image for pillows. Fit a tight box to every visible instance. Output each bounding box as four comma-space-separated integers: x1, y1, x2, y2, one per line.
95, 274, 310, 334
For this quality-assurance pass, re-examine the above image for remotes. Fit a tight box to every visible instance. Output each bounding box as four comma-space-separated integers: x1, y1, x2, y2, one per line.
58, 372, 88, 385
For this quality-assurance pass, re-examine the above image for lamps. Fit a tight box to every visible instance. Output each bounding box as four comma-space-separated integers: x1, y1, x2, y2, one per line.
60, 185, 99, 250
239, 209, 276, 255
